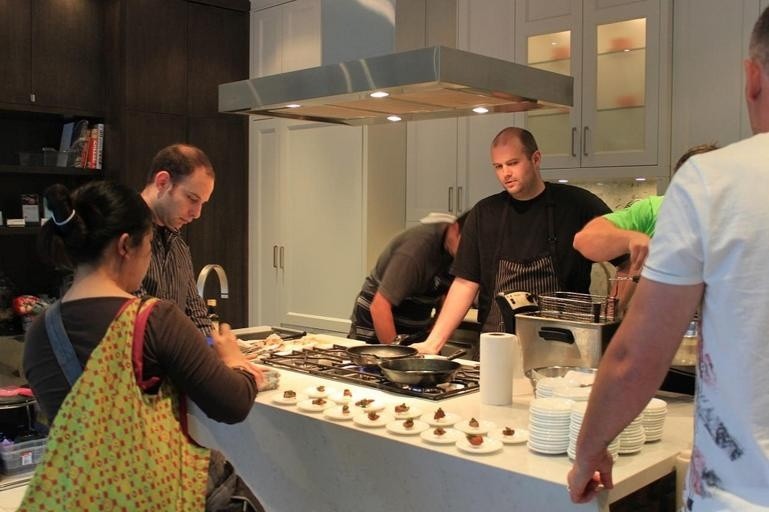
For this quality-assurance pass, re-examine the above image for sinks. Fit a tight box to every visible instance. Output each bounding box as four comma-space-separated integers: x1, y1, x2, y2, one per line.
235, 329, 284, 343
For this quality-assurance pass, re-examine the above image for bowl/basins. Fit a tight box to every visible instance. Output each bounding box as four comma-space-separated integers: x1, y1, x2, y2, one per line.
524, 364, 598, 400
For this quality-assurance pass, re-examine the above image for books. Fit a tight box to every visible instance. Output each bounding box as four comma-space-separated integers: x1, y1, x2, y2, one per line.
59, 122, 104, 171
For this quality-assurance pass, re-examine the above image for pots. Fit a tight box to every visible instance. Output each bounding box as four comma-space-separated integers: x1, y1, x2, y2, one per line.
347, 330, 419, 370
377, 348, 467, 388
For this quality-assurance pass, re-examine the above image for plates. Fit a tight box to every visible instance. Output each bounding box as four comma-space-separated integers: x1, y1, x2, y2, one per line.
270, 383, 528, 456
530, 376, 667, 464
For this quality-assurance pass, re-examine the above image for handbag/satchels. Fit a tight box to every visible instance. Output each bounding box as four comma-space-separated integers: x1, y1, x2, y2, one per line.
208, 450, 264, 511
14, 298, 211, 512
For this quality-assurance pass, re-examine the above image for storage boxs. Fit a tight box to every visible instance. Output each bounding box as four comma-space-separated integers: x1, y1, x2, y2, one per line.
1, 437, 51, 477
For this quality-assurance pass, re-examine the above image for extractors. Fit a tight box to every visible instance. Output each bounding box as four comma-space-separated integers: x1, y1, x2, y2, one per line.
218, 2, 573, 128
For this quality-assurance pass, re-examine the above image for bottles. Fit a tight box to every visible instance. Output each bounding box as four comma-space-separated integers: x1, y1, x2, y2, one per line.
206, 299, 221, 338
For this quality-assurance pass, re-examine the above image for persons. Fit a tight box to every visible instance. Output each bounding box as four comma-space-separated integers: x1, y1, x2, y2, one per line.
345, 209, 477, 358
405, 125, 629, 356
140, 145, 264, 387
568, 12, 769, 511
22, 178, 267, 510
572, 143, 720, 318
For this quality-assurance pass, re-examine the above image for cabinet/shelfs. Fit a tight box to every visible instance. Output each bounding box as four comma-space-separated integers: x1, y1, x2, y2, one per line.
510, 1, 672, 180
0, 109, 104, 338
104, 105, 249, 328
249, 0, 394, 339
103, 0, 245, 112
1, 1, 103, 117
402, 2, 515, 229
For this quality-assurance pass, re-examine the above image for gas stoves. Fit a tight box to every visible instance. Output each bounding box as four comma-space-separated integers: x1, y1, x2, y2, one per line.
259, 343, 481, 401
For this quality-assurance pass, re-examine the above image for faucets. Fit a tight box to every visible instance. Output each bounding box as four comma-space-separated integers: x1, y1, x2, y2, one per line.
196, 262, 230, 301
